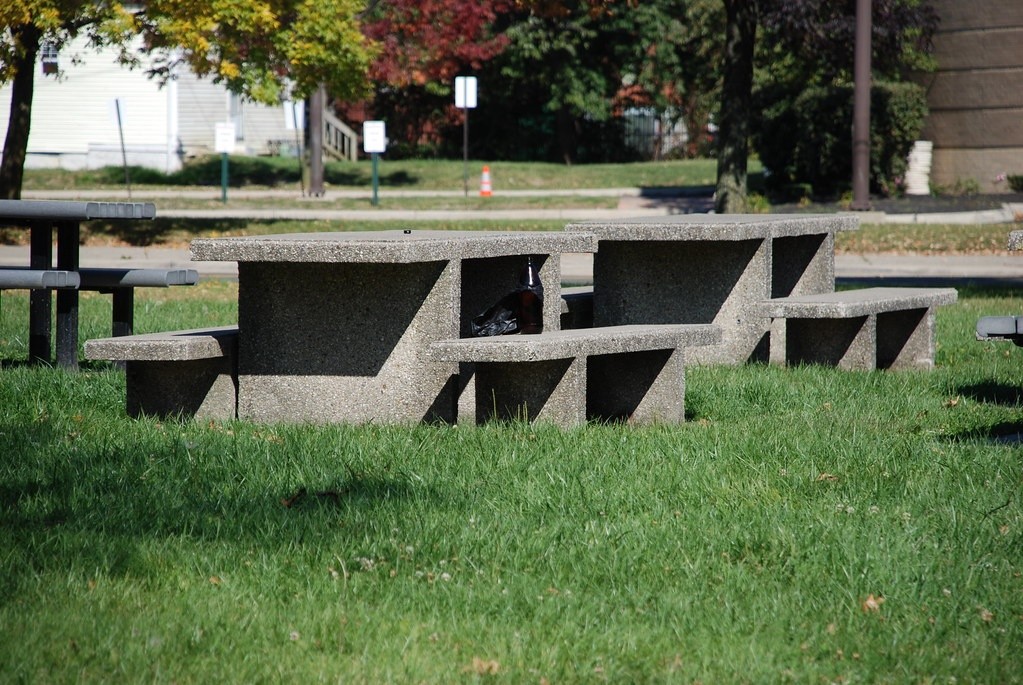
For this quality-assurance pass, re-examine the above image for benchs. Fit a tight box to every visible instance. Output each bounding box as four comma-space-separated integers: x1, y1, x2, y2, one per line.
83, 325, 239, 424
757, 286, 959, 372
0, 269, 81, 290
428, 324, 723, 431
560, 293, 592, 329
0, 266, 199, 372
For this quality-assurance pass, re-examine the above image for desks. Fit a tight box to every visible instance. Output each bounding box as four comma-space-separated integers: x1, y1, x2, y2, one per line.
188, 229, 599, 425
0, 199, 156, 371
564, 213, 863, 367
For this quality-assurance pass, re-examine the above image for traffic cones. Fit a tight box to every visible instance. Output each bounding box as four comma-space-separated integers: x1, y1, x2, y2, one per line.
479, 164, 494, 198
